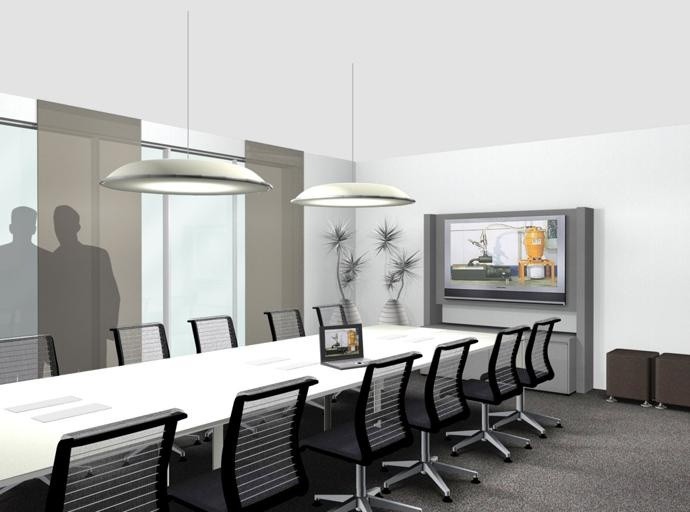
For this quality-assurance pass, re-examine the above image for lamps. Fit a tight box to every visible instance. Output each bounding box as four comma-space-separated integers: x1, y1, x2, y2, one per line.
99, 12, 270, 195
290, 64, 413, 208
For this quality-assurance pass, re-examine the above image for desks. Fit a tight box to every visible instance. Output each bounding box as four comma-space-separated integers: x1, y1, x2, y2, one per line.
0, 324, 530, 511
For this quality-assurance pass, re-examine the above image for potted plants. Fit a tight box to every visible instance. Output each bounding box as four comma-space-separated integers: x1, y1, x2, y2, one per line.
374, 216, 421, 325
321, 217, 363, 326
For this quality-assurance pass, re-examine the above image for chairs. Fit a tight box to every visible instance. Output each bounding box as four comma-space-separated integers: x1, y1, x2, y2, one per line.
0, 334, 95, 487
46, 408, 187, 511
300, 351, 427, 512
187, 315, 263, 441
438, 325, 534, 464
163, 376, 318, 510
108, 322, 201, 466
261, 308, 326, 418
380, 338, 482, 502
480, 317, 563, 439
312, 305, 372, 402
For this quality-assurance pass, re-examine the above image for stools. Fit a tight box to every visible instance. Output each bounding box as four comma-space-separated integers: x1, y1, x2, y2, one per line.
654, 353, 690, 409
606, 349, 659, 408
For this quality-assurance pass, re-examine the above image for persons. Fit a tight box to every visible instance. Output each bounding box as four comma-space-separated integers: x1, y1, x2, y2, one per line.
0, 205, 52, 383
44, 205, 121, 376
